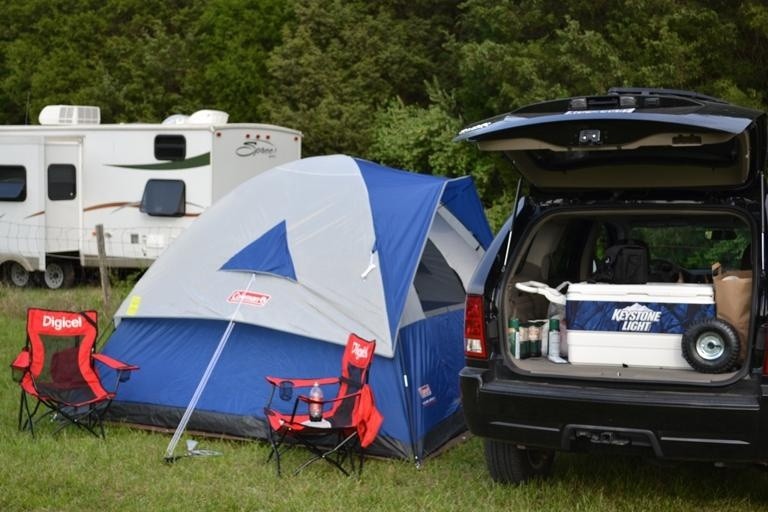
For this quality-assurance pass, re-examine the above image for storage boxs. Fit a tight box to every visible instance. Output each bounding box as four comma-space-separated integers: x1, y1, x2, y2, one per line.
566, 283, 715, 370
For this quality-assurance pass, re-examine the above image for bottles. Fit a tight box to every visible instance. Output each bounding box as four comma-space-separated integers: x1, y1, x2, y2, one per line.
308, 382, 324, 422
509, 318, 520, 360
520, 318, 545, 359
547, 319, 561, 358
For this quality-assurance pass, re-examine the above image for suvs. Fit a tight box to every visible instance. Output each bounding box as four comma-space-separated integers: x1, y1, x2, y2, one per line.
451, 86, 767, 485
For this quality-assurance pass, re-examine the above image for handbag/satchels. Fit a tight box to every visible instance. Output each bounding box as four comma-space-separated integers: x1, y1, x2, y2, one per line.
712, 263, 752, 364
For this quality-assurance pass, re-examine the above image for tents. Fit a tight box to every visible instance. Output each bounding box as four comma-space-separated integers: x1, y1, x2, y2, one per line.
47, 152, 499, 473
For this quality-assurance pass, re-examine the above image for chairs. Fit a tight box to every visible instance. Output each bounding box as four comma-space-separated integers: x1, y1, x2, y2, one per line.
264, 334, 375, 477
10, 308, 140, 439
